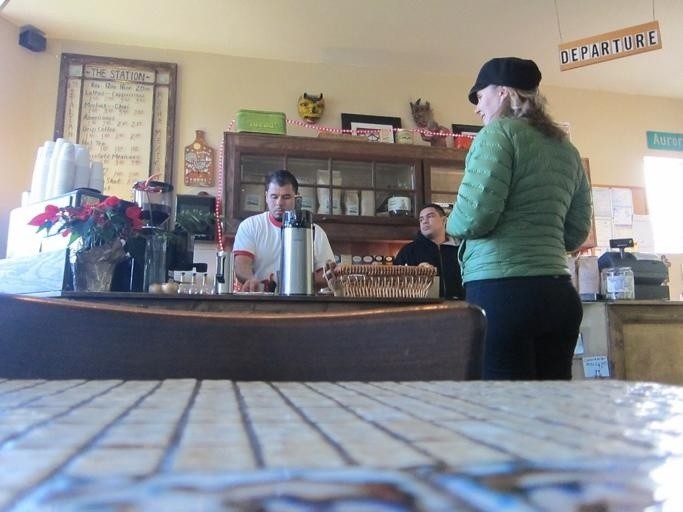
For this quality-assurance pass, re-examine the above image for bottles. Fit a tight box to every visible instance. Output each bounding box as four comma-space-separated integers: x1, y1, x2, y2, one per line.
178, 271, 210, 295
600, 266, 635, 300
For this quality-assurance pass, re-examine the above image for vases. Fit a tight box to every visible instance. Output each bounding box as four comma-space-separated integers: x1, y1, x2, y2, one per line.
67, 240, 126, 292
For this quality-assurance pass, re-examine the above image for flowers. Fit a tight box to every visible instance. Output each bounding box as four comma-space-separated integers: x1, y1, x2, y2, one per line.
28, 197, 145, 251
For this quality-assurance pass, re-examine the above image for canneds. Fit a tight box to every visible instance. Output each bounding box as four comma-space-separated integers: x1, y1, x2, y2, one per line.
395, 129, 414, 145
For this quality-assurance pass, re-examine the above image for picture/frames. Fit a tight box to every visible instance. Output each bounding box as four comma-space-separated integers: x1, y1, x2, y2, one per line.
452, 125, 484, 139
172, 195, 216, 241
341, 113, 401, 145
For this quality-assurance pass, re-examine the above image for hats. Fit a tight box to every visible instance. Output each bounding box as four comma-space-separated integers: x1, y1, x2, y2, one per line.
468, 57, 542, 105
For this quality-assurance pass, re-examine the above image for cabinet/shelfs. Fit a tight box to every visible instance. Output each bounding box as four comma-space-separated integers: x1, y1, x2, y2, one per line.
222, 130, 467, 241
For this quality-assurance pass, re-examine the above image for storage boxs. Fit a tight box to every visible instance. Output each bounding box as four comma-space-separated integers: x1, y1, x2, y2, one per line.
237, 110, 287, 137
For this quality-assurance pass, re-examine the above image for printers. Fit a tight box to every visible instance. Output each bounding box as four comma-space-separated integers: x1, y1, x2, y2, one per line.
598, 250, 671, 300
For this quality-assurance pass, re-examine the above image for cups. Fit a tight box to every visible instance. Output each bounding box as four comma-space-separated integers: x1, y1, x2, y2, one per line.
28, 137, 106, 205
278, 209, 316, 297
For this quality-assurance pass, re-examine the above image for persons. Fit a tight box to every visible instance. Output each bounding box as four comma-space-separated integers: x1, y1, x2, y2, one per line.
391, 203, 466, 300
229, 168, 335, 294
444, 57, 593, 381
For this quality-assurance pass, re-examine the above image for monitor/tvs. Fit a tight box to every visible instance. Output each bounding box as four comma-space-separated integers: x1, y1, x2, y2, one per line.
439, 243, 466, 300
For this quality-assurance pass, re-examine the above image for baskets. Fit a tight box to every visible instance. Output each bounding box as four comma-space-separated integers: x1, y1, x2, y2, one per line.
322, 260, 438, 299
